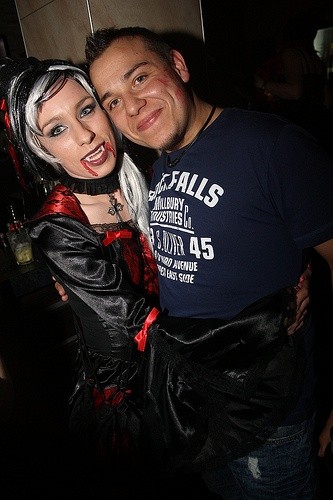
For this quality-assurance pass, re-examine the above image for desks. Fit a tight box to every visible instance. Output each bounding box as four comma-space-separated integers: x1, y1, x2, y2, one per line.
2, 244, 81, 377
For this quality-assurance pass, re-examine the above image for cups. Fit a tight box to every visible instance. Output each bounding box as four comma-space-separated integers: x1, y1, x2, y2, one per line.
6, 228, 33, 265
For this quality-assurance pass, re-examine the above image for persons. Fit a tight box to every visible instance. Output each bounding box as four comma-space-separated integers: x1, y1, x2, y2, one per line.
83, 26, 333, 499
1, 56, 158, 497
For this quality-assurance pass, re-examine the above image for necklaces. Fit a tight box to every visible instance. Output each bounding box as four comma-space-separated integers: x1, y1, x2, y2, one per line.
59, 170, 123, 225
167, 101, 219, 168
